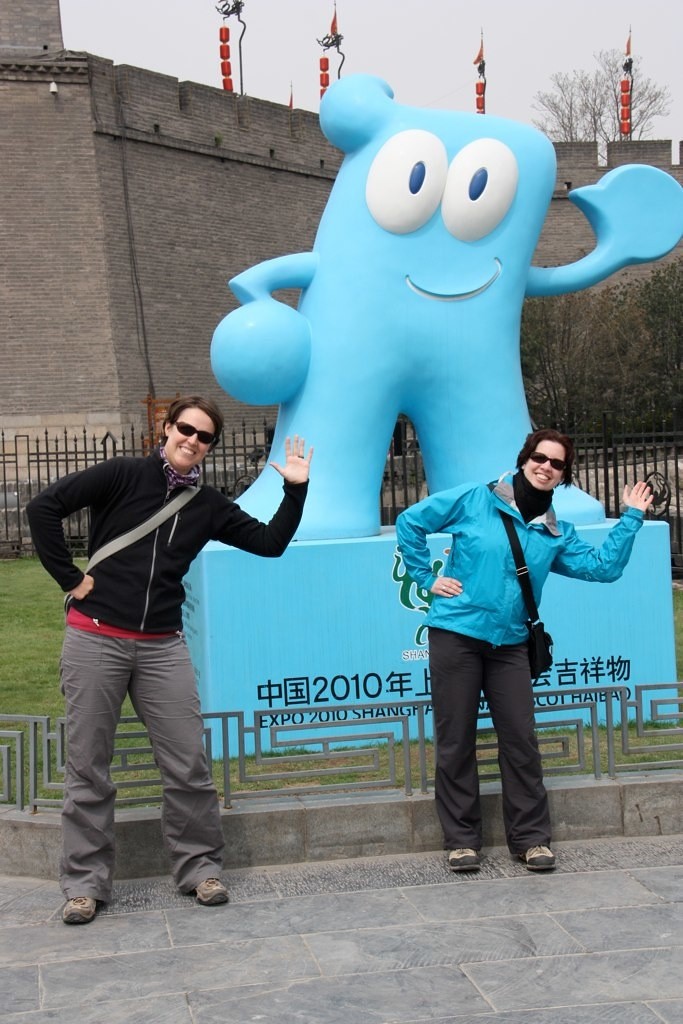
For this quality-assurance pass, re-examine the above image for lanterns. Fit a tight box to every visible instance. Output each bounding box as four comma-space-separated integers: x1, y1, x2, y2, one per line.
616, 79, 633, 138
316, 57, 332, 104
473, 80, 486, 115
219, 18, 235, 95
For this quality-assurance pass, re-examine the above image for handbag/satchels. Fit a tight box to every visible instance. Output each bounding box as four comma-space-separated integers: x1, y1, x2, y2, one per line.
526, 619, 554, 679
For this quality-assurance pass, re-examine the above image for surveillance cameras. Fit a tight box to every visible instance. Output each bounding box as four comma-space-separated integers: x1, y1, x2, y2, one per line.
50, 81, 58, 95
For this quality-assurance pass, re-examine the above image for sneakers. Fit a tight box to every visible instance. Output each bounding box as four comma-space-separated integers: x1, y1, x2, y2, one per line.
518, 845, 556, 870
194, 878, 229, 906
448, 848, 481, 871
62, 896, 97, 923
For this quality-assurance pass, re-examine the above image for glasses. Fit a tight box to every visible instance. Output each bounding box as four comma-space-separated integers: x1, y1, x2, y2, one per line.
175, 421, 216, 444
530, 451, 567, 471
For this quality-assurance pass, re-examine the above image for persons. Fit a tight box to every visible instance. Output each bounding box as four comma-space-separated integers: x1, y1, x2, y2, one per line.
391, 430, 657, 872
24, 396, 318, 925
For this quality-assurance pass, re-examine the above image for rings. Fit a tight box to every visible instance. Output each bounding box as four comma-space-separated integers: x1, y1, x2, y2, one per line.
298, 455, 305, 459
441, 586, 446, 591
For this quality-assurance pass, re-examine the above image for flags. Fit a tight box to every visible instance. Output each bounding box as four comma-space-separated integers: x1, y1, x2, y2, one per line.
472, 44, 484, 65
624, 35, 632, 56
327, 10, 338, 36
